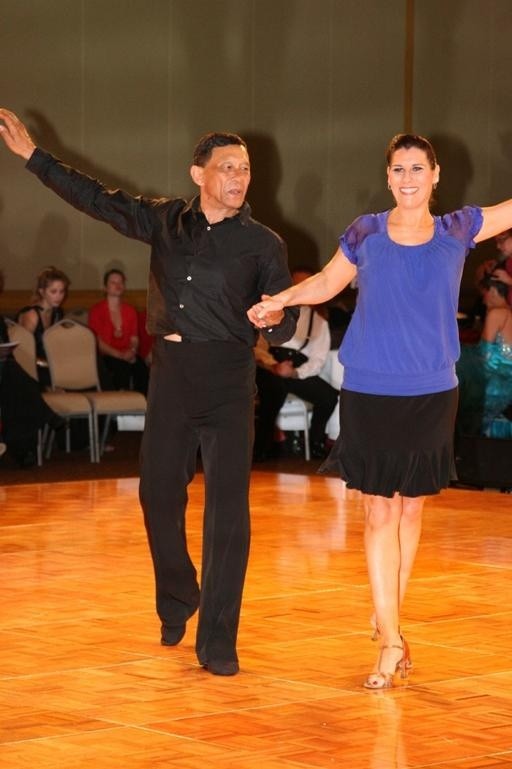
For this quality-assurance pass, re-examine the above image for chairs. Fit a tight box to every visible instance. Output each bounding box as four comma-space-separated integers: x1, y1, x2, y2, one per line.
43, 318, 148, 462
5, 317, 95, 467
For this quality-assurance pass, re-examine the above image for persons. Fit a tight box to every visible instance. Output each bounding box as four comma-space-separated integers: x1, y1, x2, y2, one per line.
253, 266, 338, 464
0, 106, 302, 677
2, 267, 154, 470
450, 227, 511, 442
252, 133, 512, 690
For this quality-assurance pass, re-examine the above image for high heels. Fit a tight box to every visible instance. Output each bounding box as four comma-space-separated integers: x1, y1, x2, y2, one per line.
364, 615, 412, 689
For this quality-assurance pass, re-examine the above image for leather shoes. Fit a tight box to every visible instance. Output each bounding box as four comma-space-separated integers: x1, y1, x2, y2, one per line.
203, 661, 239, 675
161, 622, 185, 645
